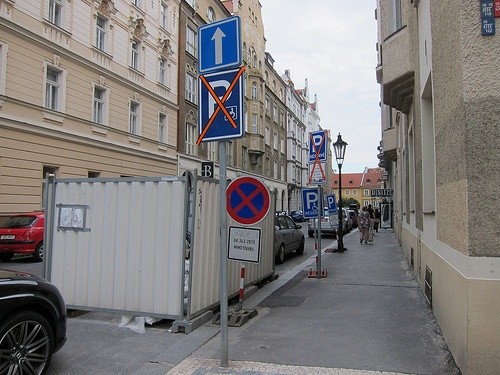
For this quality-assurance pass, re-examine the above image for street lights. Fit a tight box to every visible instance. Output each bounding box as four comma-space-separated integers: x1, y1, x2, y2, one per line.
332, 132, 349, 252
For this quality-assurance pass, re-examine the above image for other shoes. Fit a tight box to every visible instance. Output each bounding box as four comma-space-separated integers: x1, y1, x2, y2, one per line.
360, 238, 363, 243
364, 240, 367, 244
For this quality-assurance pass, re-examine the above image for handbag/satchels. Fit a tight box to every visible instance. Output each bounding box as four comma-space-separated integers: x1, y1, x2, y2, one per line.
368, 226, 374, 242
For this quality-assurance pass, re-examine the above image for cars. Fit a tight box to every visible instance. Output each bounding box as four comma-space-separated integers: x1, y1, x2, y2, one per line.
0, 209, 45, 262
287, 210, 309, 222
308, 203, 359, 238
274, 210, 305, 264
0, 265, 68, 375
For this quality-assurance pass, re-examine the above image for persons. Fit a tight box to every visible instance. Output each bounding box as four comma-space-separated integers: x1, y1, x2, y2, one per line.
357, 203, 381, 244
